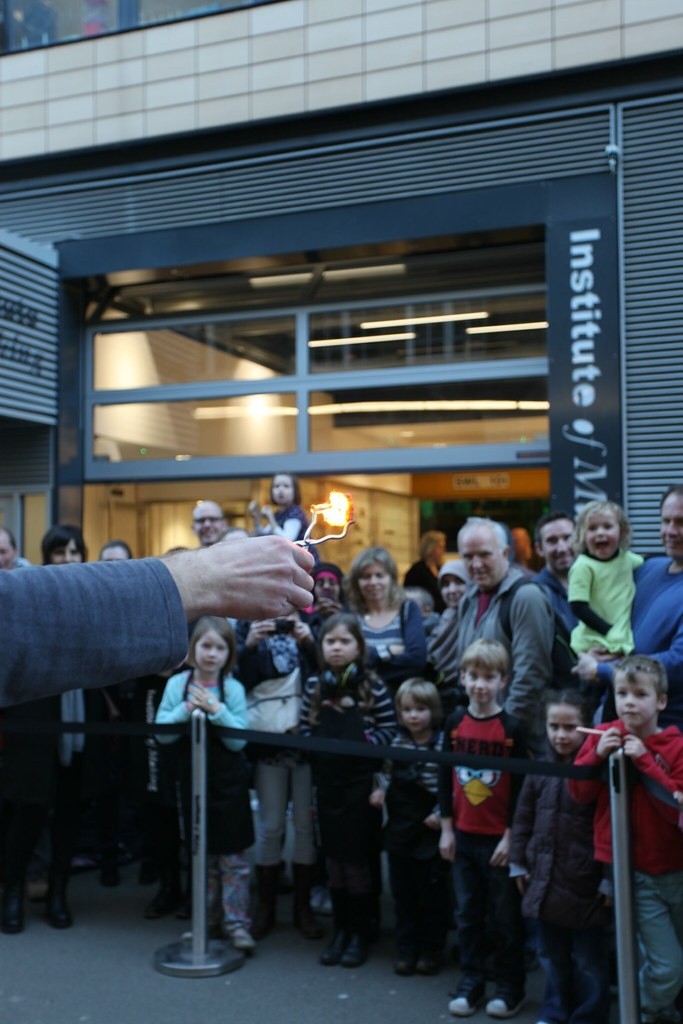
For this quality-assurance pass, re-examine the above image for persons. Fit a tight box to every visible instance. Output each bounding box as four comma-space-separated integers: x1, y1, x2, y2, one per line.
0, 535, 314, 707
0, 524, 194, 935
154, 474, 683, 1024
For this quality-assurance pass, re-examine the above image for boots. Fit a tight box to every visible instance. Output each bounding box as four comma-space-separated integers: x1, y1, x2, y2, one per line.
45, 868, 72, 926
292, 861, 320, 937
248, 864, 281, 939
0, 867, 25, 933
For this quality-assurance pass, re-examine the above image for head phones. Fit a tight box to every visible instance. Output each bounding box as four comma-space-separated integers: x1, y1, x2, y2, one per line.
316, 659, 366, 697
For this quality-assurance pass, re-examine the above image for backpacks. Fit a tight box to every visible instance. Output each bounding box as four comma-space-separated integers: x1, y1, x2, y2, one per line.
501, 566, 578, 689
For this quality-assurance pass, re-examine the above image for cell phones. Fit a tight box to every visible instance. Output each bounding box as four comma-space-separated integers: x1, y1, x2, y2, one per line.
319, 589, 336, 603
267, 619, 296, 635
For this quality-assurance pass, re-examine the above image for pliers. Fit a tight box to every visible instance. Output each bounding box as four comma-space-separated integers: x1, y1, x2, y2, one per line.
292, 503, 356, 552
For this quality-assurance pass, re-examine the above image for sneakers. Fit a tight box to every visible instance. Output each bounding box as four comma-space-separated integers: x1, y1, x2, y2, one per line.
339, 936, 376, 966
320, 933, 349, 966
448, 976, 485, 1015
485, 979, 529, 1018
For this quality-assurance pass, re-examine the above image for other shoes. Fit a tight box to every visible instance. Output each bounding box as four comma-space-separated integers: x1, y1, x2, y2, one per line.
139, 860, 159, 884
415, 952, 442, 973
100, 862, 119, 887
394, 951, 416, 974
175, 896, 192, 919
145, 884, 176, 917
229, 927, 254, 947
